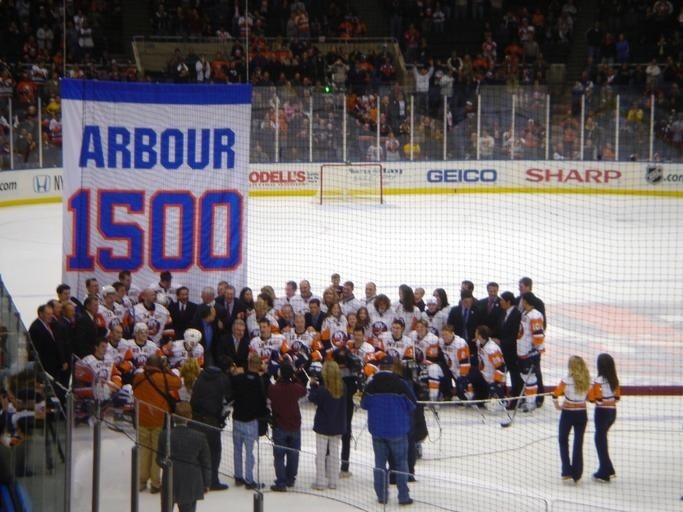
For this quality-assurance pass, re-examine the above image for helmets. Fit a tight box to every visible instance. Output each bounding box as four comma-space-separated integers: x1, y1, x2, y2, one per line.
426, 296, 438, 305
102, 284, 116, 297
183, 328, 203, 344
133, 322, 149, 336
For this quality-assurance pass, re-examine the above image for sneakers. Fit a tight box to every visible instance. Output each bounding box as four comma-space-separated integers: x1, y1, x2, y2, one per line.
150, 484, 161, 493
246, 482, 266, 490
273, 476, 296, 487
593, 472, 610, 481
234, 477, 245, 486
311, 482, 326, 490
210, 482, 230, 491
607, 468, 616, 475
270, 484, 287, 492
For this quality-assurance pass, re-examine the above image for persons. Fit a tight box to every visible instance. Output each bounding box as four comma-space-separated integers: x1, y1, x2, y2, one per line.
445, 290, 483, 358
257, 293, 279, 320
401, 345, 444, 413
156, 401, 212, 512
118, 269, 131, 292
587, 353, 621, 483
112, 282, 135, 320
323, 273, 344, 309
77, 297, 107, 335
0, 0, 683, 170
105, 323, 128, 359
303, 299, 325, 332
459, 278, 478, 310
280, 280, 297, 304
261, 285, 283, 311
238, 287, 257, 312
82, 278, 102, 305
132, 354, 182, 494
217, 281, 228, 297
493, 291, 522, 410
289, 280, 322, 315
433, 287, 453, 317
275, 304, 295, 329
515, 291, 547, 414
396, 284, 420, 334
308, 361, 348, 490
75, 337, 133, 430
98, 290, 125, 326
338, 280, 361, 316
421, 294, 449, 338
225, 353, 270, 489
50, 300, 63, 321
321, 286, 350, 315
345, 326, 375, 361
54, 284, 82, 309
319, 303, 350, 342
514, 276, 547, 409
406, 319, 438, 363
214, 319, 250, 375
438, 325, 472, 408
60, 303, 77, 333
247, 299, 278, 333
128, 322, 157, 367
474, 282, 504, 339
198, 289, 226, 323
361, 282, 377, 304
190, 356, 229, 491
354, 307, 373, 339
151, 270, 176, 308
360, 354, 417, 504
414, 287, 426, 312
267, 364, 305, 490
169, 328, 204, 375
377, 317, 412, 362
213, 286, 244, 327
474, 324, 514, 428
387, 366, 425, 483
282, 315, 324, 353
247, 318, 286, 358
345, 312, 358, 336
168, 287, 198, 325
551, 356, 592, 483
29, 304, 71, 388
132, 287, 172, 348
365, 294, 396, 330
148, 336, 175, 371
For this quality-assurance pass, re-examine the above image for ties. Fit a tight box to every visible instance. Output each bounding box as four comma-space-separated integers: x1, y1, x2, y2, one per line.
181, 303, 184, 313
463, 308, 468, 322
234, 337, 239, 353
226, 302, 230, 319
